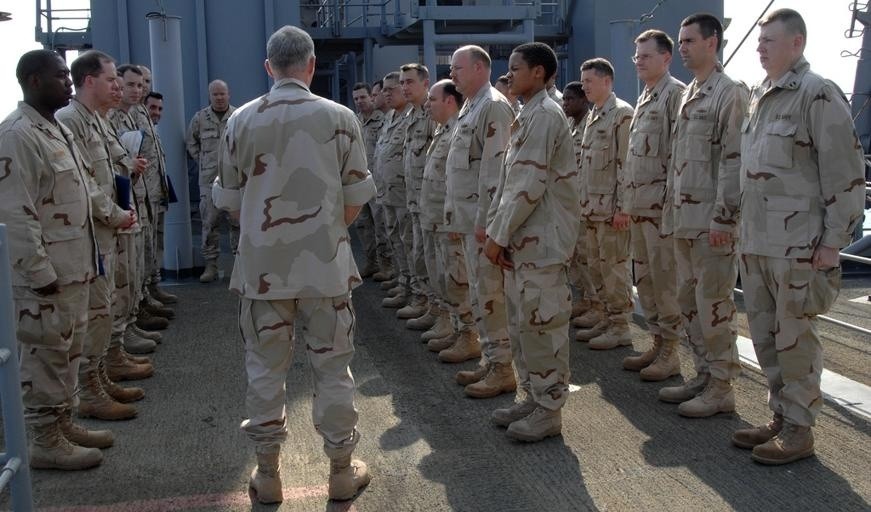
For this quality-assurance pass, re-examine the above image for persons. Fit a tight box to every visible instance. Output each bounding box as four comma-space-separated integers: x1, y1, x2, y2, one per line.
211, 26, 376, 503
186, 79, 239, 281
351, 42, 580, 440
734, 8, 866, 466
0, 50, 179, 473
563, 12, 750, 417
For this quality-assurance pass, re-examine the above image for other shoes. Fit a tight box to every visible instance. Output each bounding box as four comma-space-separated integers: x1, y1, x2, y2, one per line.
380, 280, 394, 288
387, 285, 401, 294
373, 271, 392, 280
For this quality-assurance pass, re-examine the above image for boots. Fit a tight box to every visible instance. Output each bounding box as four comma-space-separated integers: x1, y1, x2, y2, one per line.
678, 377, 736, 417
421, 310, 451, 343
199, 256, 220, 283
576, 311, 610, 341
506, 405, 563, 441
325, 454, 373, 504
464, 362, 516, 398
427, 331, 460, 351
491, 399, 539, 426
623, 336, 663, 370
749, 421, 814, 465
248, 451, 284, 507
27, 280, 180, 474
406, 304, 441, 330
382, 290, 411, 306
641, 339, 680, 381
397, 293, 428, 319
659, 372, 710, 402
438, 331, 481, 363
454, 356, 491, 386
572, 298, 591, 313
589, 323, 632, 350
572, 301, 607, 327
731, 415, 783, 449
